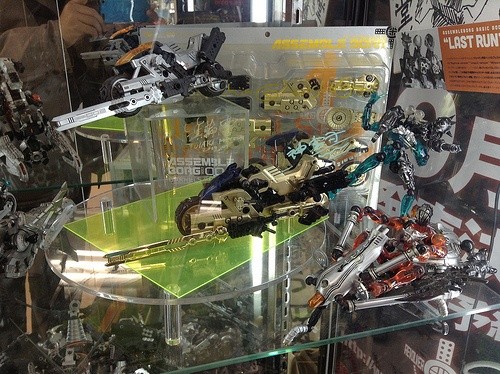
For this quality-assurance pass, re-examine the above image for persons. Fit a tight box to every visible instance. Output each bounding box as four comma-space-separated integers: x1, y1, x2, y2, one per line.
0, 1, 170, 351
